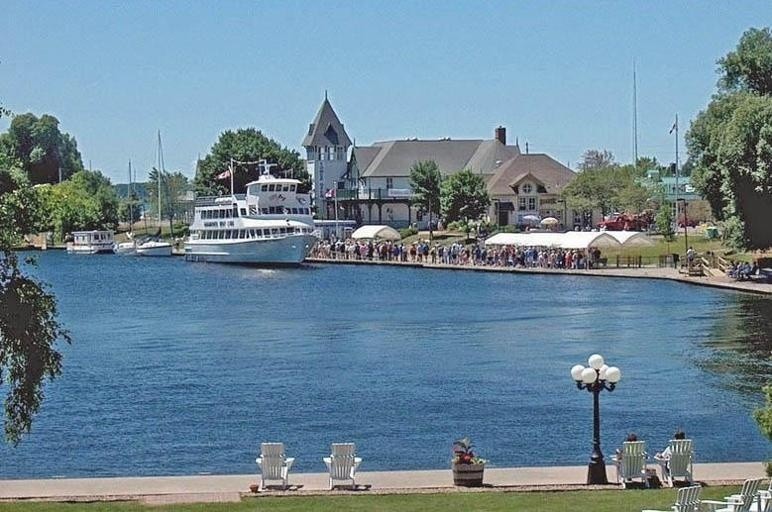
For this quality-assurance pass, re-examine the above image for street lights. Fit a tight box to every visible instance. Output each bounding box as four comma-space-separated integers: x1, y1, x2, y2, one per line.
569, 353, 621, 486
558, 198, 567, 225
676, 197, 688, 254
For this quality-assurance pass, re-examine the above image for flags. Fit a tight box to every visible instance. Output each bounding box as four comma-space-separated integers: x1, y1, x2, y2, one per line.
217, 170, 230, 179
325, 188, 335, 198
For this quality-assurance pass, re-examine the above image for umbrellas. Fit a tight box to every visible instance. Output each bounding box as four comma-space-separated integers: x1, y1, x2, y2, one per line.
541, 217, 558, 225
523, 215, 539, 230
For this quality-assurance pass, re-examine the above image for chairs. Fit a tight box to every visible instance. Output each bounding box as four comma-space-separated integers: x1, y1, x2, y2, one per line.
253, 441, 295, 493
609, 440, 772, 512
322, 441, 364, 490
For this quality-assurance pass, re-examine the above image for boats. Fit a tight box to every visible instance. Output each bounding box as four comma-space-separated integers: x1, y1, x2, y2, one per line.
64, 229, 116, 254
182, 159, 318, 267
112, 237, 174, 258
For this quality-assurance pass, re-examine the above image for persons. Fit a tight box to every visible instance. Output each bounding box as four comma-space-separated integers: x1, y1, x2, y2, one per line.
687, 246, 696, 267
655, 432, 685, 481
625, 433, 638, 481
386, 208, 394, 220
728, 260, 758, 281
308, 237, 602, 269
